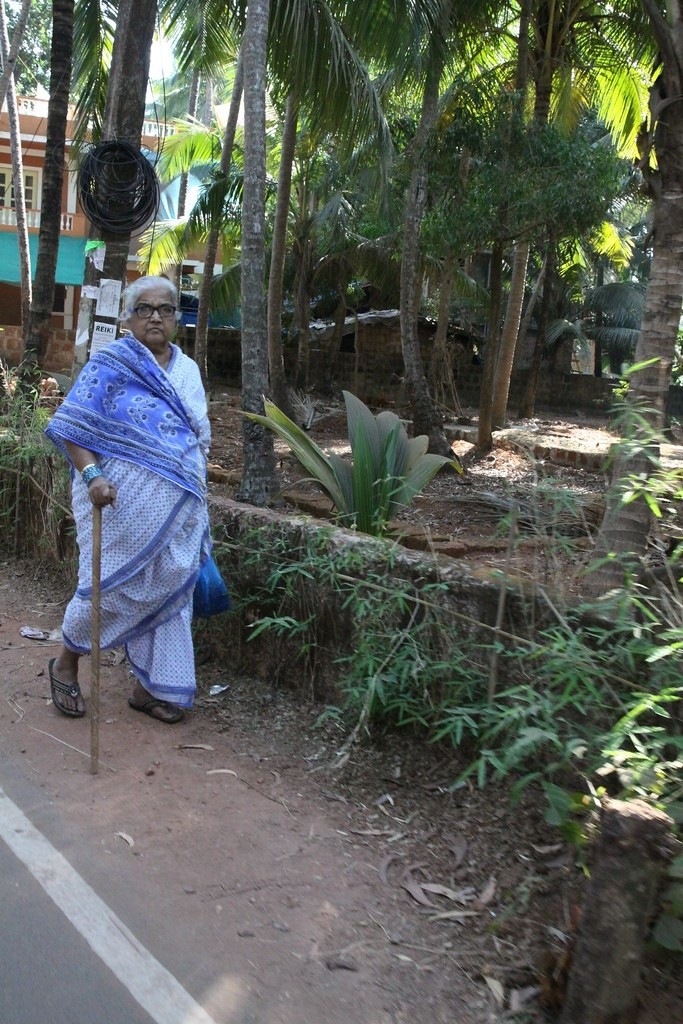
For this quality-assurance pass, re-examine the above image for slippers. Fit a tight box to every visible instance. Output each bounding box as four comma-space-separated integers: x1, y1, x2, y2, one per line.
127, 695, 184, 723
48, 657, 86, 717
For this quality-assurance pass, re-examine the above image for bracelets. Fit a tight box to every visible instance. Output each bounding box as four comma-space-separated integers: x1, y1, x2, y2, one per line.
80, 464, 104, 488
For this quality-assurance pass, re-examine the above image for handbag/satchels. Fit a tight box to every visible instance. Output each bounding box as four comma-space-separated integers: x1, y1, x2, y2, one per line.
192, 554, 231, 618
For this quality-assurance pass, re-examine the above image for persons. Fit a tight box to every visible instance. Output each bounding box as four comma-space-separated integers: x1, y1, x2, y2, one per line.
45, 276, 232, 724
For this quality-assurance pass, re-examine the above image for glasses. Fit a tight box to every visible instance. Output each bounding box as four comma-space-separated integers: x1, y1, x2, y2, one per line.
131, 303, 177, 319
